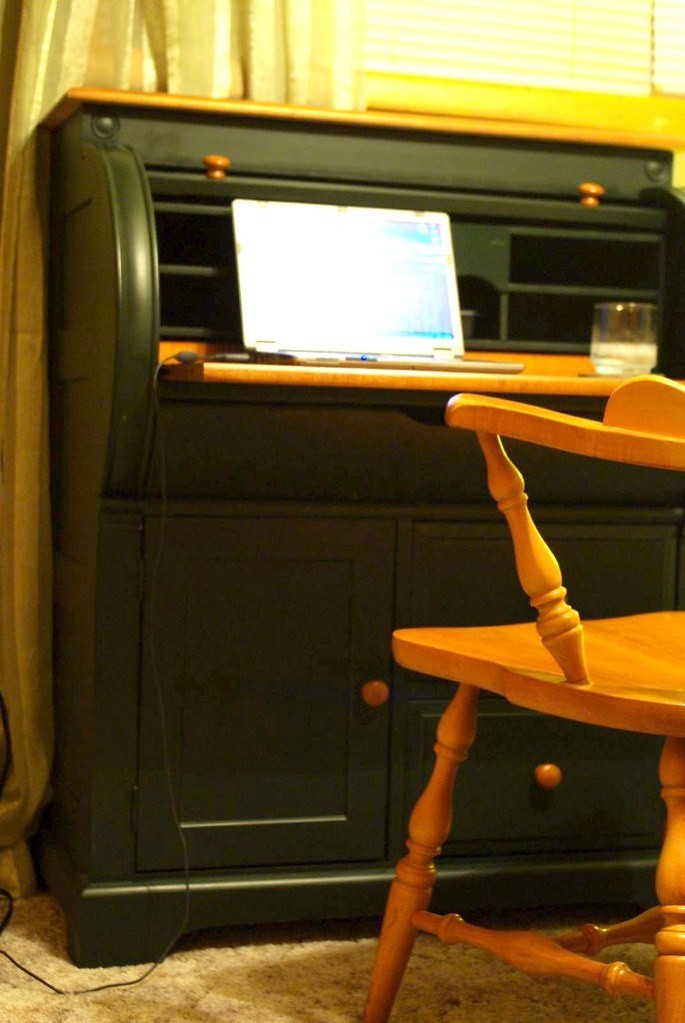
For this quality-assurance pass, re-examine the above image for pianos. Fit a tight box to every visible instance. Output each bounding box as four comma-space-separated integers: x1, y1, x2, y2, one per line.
36, 66, 685, 970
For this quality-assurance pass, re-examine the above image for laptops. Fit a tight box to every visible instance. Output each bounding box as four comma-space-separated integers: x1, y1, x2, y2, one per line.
231, 196, 525, 377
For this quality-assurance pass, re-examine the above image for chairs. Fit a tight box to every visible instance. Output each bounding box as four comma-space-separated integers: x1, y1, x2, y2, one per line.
364, 372, 685, 1022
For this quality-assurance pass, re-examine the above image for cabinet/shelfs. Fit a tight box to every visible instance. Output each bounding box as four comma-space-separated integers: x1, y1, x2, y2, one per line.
32, 87, 685, 969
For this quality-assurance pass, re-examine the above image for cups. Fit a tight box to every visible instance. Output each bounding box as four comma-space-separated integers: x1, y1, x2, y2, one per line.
589, 298, 657, 379
